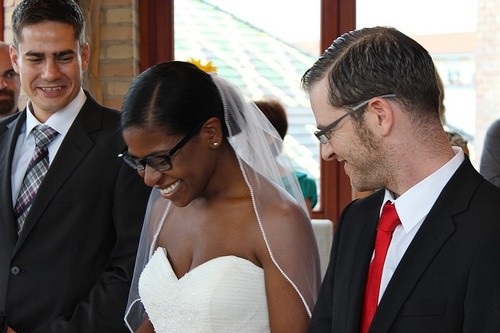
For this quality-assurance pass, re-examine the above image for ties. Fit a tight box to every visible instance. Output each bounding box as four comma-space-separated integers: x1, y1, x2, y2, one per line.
360, 200, 402, 333
13, 125, 60, 242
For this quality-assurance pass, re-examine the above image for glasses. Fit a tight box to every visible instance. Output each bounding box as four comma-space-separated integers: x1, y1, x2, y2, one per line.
313, 94, 397, 144
120, 126, 201, 172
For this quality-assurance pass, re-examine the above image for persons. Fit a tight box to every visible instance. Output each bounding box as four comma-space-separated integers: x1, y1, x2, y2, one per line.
302, 26, 500, 333
122, 61, 320, 332
0, 0, 156, 333
479, 120, 500, 188
0, 40, 23, 121
249, 98, 318, 222
